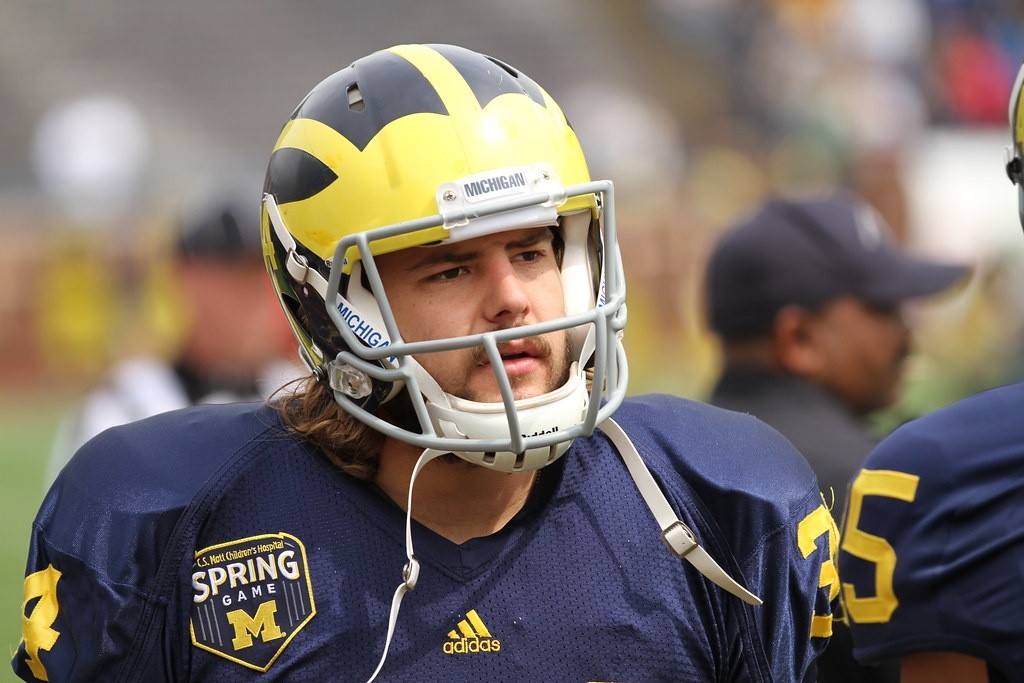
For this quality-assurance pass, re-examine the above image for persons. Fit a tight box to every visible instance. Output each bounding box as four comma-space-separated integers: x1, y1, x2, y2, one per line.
12, 43, 852, 683
702, 62, 1024, 683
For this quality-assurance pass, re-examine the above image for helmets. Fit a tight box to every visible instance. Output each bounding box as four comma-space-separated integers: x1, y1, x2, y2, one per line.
260, 44, 629, 473
1006, 63, 1024, 233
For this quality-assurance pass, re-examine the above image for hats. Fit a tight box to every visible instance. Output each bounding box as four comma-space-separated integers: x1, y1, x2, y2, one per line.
704, 187, 976, 335
173, 203, 263, 264
